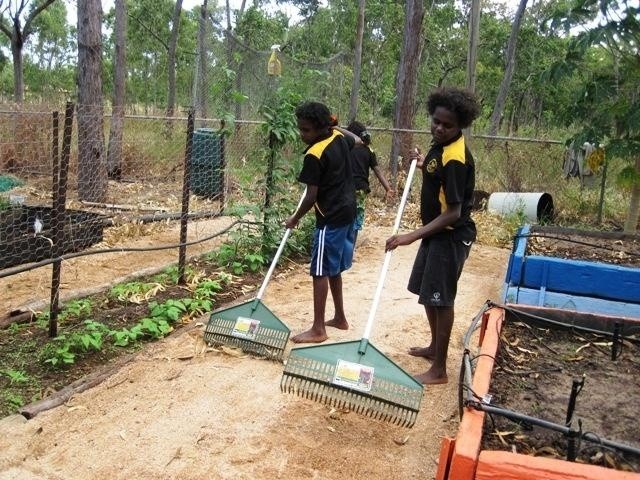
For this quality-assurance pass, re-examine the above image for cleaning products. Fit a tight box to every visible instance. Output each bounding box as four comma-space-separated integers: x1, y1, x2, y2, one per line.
266, 44, 282, 77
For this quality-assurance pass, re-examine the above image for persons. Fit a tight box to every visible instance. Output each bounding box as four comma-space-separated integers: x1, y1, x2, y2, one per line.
281, 99, 365, 346
384, 85, 484, 387
343, 120, 394, 232
